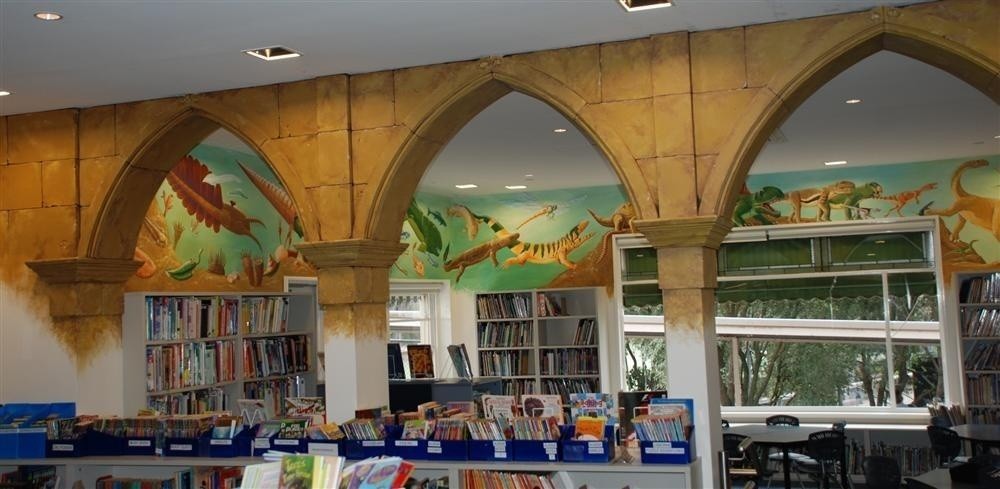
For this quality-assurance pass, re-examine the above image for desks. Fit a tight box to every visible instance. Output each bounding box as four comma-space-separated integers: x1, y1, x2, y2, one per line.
948, 423, 1000, 462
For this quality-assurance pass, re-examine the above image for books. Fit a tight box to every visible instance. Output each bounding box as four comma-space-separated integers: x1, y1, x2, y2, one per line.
99, 467, 242, 488
144, 296, 314, 415
572, 318, 596, 345
45, 414, 243, 437
478, 321, 533, 347
727, 436, 1000, 475
927, 400, 964, 426
537, 294, 560, 317
477, 294, 532, 320
481, 350, 536, 394
540, 348, 598, 405
242, 391, 692, 488
954, 270, 1000, 425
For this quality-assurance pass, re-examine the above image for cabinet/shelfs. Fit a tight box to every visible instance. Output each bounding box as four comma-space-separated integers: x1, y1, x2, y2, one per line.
954, 269, 1000, 458
472, 285, 608, 397
122, 289, 311, 421
846, 425, 946, 484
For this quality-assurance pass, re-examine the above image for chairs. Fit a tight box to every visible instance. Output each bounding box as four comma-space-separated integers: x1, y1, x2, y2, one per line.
927, 416, 960, 471
721, 415, 844, 489
